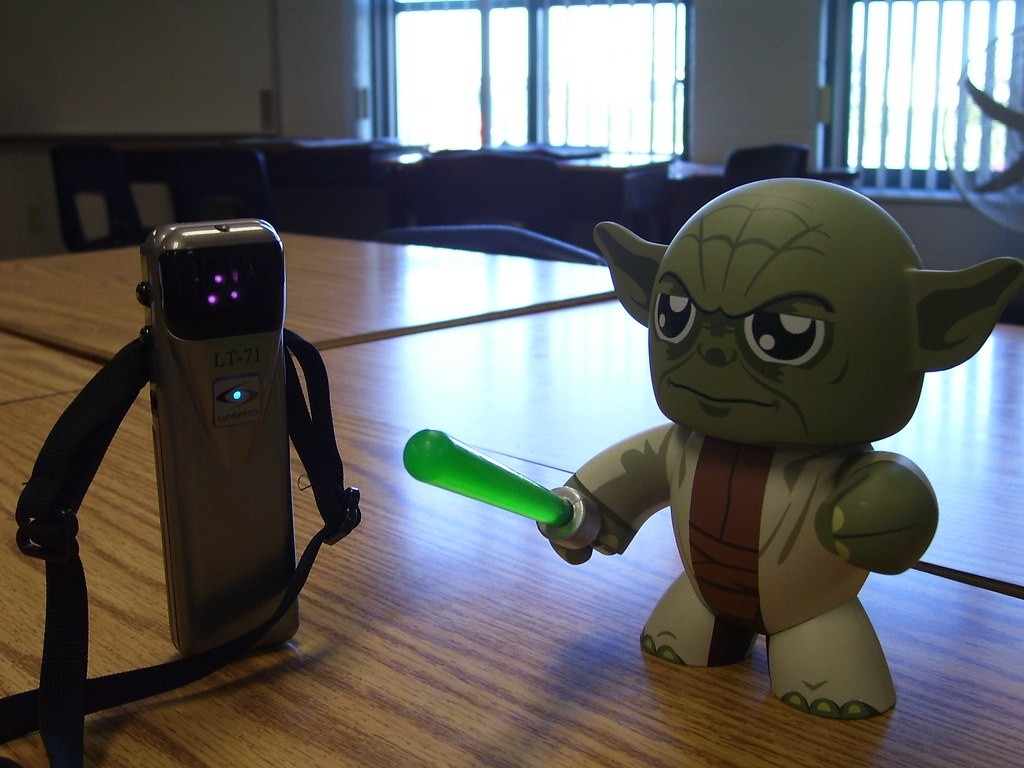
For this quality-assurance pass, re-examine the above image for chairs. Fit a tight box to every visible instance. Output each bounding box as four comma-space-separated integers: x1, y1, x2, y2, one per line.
52, 143, 148, 253
723, 145, 809, 190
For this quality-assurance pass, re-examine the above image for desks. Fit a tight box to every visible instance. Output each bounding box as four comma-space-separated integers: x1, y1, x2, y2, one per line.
384, 155, 677, 252
0, 231, 1024, 768
114, 139, 407, 231
